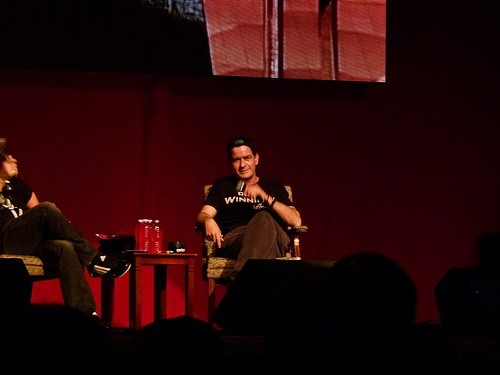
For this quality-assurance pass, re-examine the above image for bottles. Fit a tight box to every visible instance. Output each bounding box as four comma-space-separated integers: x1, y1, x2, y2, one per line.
135, 219, 162, 254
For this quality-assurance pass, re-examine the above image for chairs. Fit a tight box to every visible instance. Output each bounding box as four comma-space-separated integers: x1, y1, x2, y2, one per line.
202, 185, 307, 321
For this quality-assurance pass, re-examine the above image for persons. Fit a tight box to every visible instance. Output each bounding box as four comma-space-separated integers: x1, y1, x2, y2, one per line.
0, 146, 132, 328
197, 139, 302, 279
0, 248, 500, 375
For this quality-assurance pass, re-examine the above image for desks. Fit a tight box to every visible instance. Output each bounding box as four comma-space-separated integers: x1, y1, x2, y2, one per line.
101, 253, 198, 329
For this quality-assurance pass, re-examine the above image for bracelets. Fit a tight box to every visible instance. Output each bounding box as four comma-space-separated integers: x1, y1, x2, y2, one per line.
266, 193, 276, 208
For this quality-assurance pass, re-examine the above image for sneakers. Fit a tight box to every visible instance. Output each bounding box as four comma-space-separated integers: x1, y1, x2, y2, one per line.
88, 251, 131, 279
